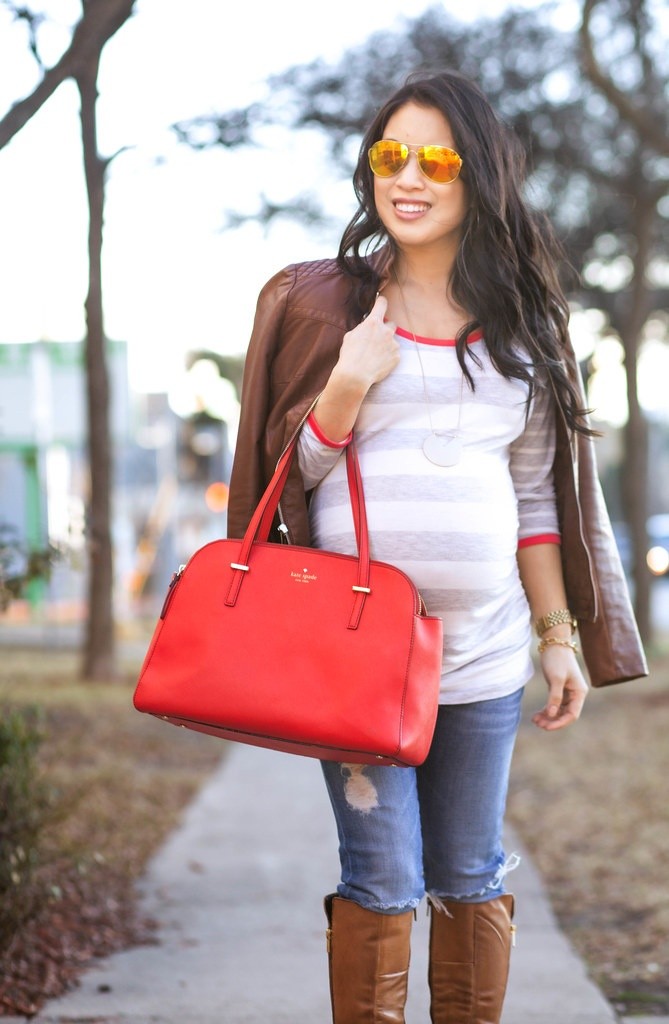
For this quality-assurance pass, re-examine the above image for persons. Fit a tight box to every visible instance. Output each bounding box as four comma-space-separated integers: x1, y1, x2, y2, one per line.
226, 72, 650, 1024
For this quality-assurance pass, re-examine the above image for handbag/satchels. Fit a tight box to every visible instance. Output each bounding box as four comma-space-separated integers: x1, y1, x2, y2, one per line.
133, 434, 443, 769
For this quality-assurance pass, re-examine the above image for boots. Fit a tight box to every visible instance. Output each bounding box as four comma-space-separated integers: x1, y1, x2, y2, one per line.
426, 893, 515, 1024
323, 892, 416, 1024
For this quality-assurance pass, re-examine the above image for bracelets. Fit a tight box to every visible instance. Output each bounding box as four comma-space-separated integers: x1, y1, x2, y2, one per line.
536, 609, 583, 653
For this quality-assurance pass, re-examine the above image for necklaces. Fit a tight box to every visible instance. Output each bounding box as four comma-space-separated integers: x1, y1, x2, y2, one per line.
398, 277, 465, 467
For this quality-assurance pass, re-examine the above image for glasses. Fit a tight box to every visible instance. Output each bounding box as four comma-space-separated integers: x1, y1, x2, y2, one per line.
368, 138, 462, 184
